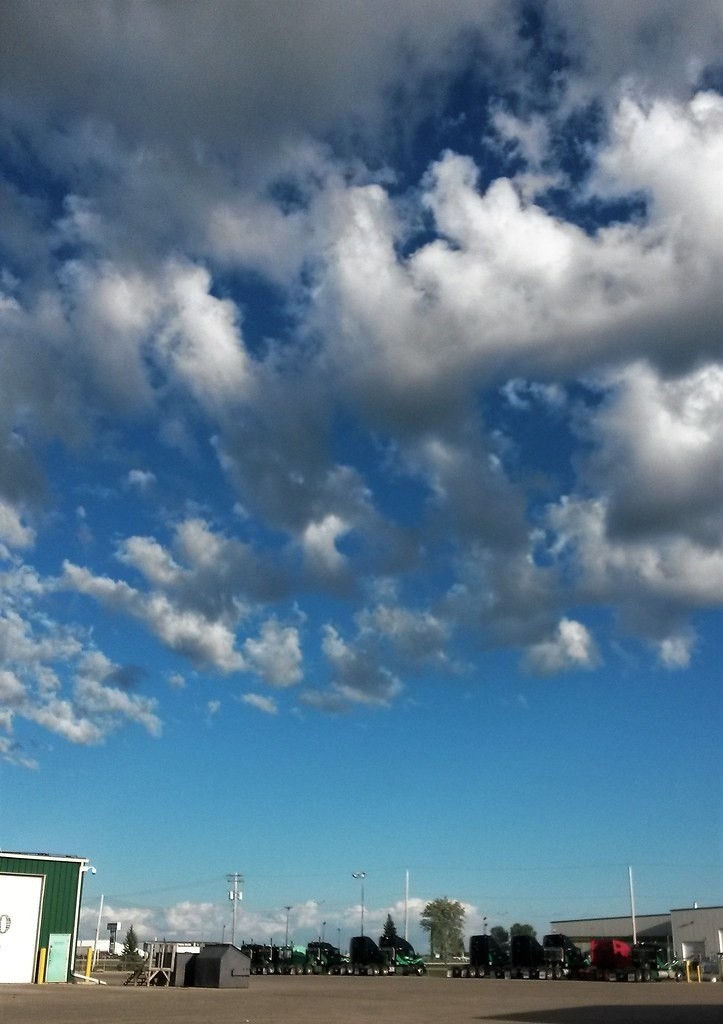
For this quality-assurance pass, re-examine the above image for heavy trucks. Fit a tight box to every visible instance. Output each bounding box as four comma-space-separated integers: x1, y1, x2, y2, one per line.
240, 933, 686, 983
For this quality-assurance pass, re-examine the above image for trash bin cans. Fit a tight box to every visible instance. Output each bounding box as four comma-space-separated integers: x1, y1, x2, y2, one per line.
193, 944, 252, 988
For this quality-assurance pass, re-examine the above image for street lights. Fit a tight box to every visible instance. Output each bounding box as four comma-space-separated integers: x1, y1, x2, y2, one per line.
483, 917, 488, 935
284, 907, 292, 946
353, 872, 366, 936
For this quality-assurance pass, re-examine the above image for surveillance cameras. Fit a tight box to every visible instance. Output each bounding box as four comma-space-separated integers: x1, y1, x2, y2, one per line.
91, 869, 96, 874
